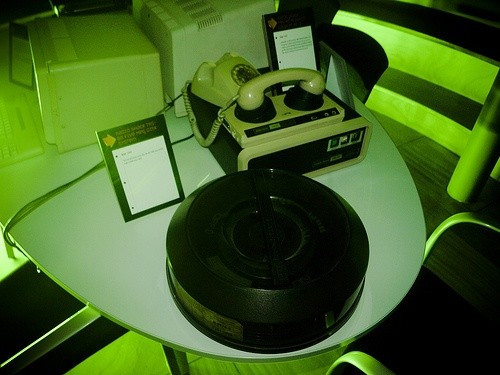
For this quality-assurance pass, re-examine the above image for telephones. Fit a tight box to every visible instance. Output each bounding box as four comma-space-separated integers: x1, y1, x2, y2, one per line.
182, 56, 372, 178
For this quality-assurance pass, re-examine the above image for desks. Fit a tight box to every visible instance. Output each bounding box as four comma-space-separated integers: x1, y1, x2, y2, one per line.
0, 94, 427, 362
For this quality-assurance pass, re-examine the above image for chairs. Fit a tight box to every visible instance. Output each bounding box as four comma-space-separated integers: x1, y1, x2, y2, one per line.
326, 0, 500, 375
